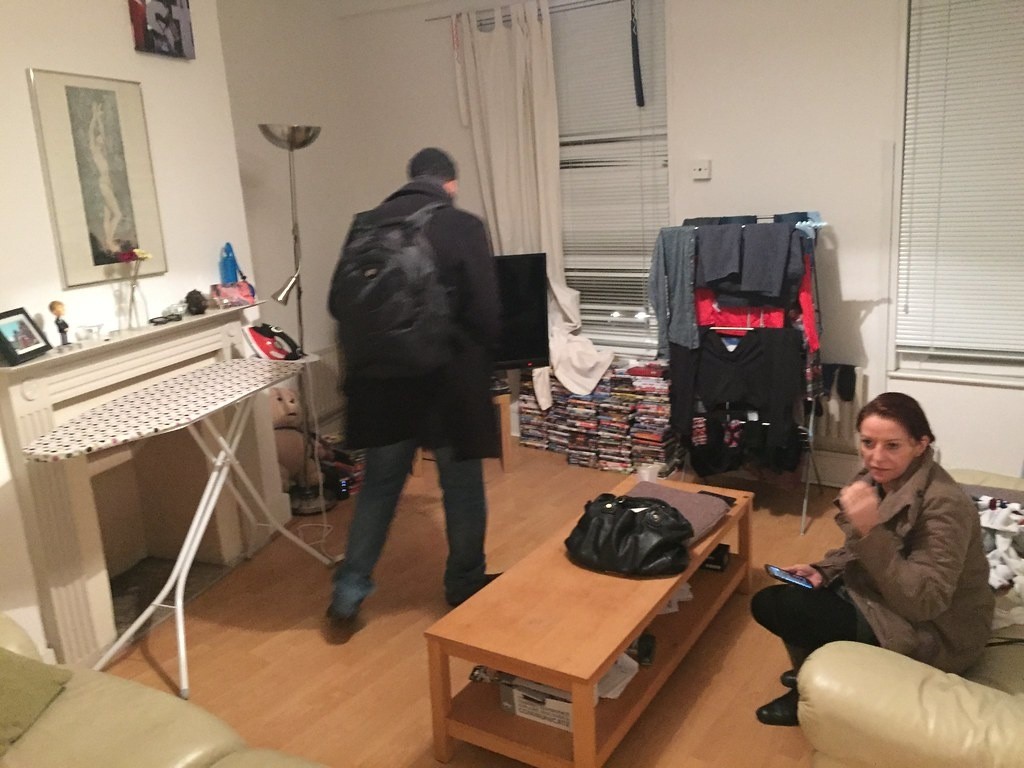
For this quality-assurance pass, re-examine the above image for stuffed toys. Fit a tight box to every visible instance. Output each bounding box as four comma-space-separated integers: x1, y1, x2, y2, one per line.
269, 386, 325, 490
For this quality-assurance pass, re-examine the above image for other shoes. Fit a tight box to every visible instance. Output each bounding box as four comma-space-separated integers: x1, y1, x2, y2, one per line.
451, 574, 504, 607
326, 602, 355, 626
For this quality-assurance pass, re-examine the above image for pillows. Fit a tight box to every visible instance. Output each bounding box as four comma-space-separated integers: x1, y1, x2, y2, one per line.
0, 648, 74, 756
622, 478, 730, 552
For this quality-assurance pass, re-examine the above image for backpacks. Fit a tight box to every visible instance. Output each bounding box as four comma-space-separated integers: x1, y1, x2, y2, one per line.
336, 201, 463, 388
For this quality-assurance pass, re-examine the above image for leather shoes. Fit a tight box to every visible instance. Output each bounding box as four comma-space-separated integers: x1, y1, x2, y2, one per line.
757, 689, 800, 726
781, 669, 798, 688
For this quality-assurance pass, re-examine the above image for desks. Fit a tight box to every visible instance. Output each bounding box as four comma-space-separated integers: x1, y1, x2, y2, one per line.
421, 469, 756, 768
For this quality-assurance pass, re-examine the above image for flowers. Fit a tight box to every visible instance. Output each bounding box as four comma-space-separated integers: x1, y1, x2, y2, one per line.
113, 243, 149, 286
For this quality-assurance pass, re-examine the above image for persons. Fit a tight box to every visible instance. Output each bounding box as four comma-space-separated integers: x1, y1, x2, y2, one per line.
326, 146, 506, 622
13, 329, 31, 348
750, 392, 996, 728
49, 300, 71, 345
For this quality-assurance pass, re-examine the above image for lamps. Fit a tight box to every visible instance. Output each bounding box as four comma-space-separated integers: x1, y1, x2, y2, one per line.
260, 122, 338, 517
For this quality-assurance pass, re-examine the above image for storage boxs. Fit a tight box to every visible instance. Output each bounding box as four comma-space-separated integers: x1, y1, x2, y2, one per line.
497, 670, 599, 734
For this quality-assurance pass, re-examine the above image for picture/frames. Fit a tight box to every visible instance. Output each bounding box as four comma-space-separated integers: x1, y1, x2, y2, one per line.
25, 66, 168, 290
0, 308, 53, 367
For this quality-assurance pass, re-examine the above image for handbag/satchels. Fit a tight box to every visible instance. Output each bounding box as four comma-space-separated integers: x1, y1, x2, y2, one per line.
564, 493, 696, 577
454, 387, 504, 462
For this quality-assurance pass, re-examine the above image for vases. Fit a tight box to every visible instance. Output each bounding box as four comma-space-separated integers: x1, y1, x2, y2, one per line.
111, 278, 149, 338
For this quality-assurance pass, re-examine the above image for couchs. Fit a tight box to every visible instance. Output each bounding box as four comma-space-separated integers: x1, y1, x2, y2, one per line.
0, 613, 336, 768
798, 466, 1024, 768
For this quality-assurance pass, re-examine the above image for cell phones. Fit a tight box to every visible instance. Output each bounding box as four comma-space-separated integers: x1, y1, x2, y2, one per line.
765, 564, 814, 590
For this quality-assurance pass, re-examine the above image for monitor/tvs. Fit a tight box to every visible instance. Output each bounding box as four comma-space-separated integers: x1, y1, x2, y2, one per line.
487, 253, 550, 371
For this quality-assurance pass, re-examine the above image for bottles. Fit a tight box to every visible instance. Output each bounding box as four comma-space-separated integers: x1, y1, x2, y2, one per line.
222, 243, 237, 284
